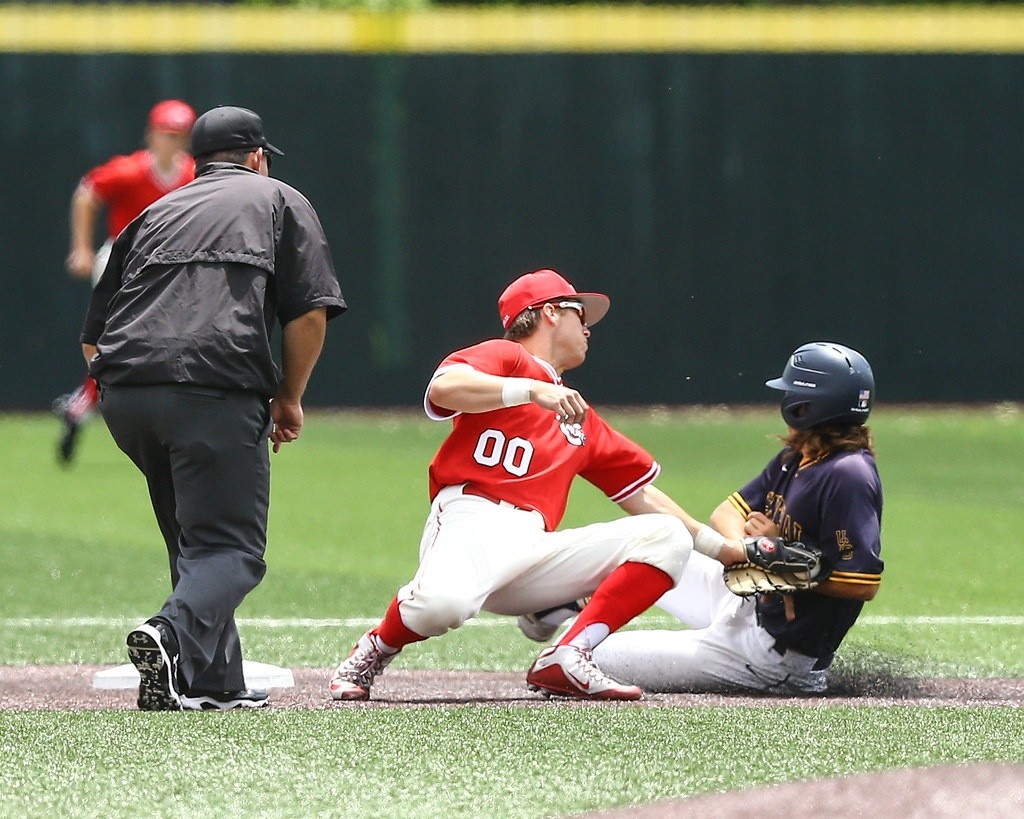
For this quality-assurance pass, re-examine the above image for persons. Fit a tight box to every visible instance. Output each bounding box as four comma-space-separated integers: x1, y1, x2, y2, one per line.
517, 338, 884, 696
89, 103, 348, 714
50, 101, 198, 465
330, 268, 832, 703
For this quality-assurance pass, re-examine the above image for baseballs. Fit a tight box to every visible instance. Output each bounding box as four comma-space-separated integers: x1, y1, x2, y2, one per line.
793, 562, 821, 580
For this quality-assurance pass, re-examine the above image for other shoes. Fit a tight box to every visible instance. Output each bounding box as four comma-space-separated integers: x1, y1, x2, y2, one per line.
516, 613, 558, 642
57, 409, 82, 462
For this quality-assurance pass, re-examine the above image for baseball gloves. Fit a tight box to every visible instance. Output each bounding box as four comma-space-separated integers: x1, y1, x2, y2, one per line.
723, 535, 832, 596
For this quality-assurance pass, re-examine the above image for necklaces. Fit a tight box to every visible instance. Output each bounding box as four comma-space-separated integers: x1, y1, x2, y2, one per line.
531, 354, 563, 387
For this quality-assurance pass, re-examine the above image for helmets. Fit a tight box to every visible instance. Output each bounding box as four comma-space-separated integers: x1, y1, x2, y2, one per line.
765, 342, 875, 430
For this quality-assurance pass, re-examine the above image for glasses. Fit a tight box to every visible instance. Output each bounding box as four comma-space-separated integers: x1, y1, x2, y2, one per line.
527, 301, 587, 326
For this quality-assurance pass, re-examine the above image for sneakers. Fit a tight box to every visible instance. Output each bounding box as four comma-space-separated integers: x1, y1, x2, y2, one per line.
526, 624, 642, 701
126, 621, 184, 712
328, 630, 402, 701
178, 686, 270, 712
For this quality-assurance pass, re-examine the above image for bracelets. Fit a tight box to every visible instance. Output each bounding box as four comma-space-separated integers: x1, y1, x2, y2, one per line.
500, 371, 533, 411
692, 522, 729, 561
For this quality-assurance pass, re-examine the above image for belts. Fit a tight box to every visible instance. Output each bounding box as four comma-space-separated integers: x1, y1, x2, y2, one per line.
773, 644, 832, 671
462, 485, 517, 509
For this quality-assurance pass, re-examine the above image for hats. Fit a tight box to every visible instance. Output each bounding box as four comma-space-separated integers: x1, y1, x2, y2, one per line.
497, 269, 610, 330
148, 100, 197, 136
191, 105, 285, 159
